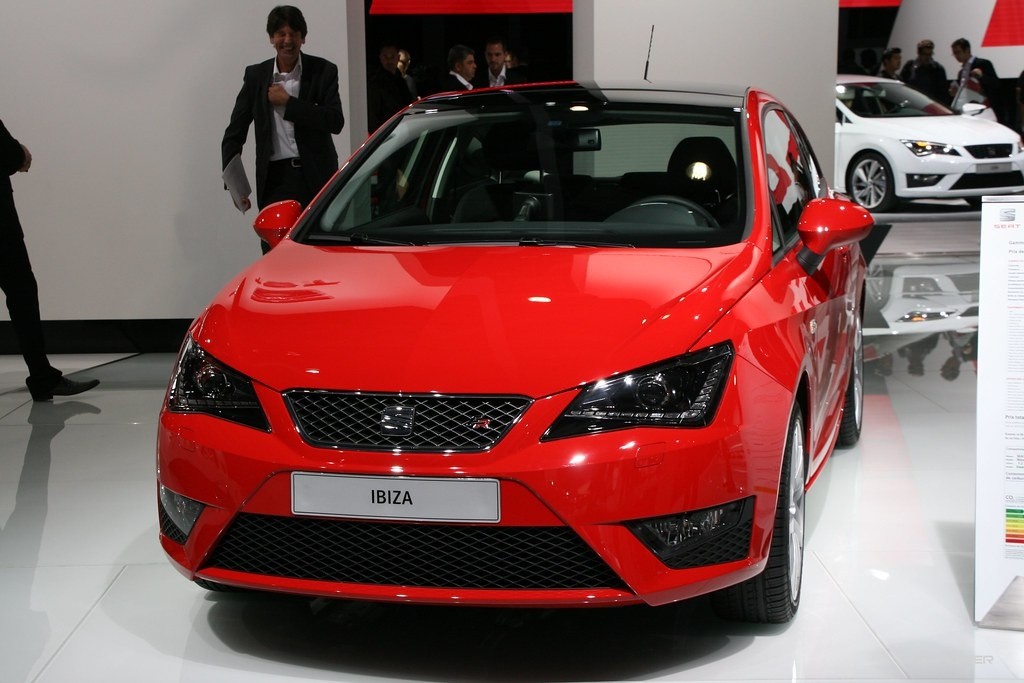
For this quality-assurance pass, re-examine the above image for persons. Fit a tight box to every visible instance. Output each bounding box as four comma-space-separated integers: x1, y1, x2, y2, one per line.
367, 42, 422, 210
949, 39, 1006, 122
900, 40, 947, 103
0, 119, 100, 401
869, 48, 904, 114
221, 5, 344, 256
441, 39, 531, 214
1016, 72, 1024, 126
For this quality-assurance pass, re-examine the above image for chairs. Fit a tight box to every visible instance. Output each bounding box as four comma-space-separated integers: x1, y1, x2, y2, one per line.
452, 121, 579, 222
658, 134, 737, 210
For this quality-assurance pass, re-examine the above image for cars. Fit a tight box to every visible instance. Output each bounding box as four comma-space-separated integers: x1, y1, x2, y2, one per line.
155, 83, 870, 628
835, 77, 1024, 212
856, 215, 981, 336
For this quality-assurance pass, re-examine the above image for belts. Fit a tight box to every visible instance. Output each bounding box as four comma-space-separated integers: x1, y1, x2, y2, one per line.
269, 157, 304, 168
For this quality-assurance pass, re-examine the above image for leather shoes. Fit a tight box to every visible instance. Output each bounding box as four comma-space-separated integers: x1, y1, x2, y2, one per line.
31, 374, 100, 401
